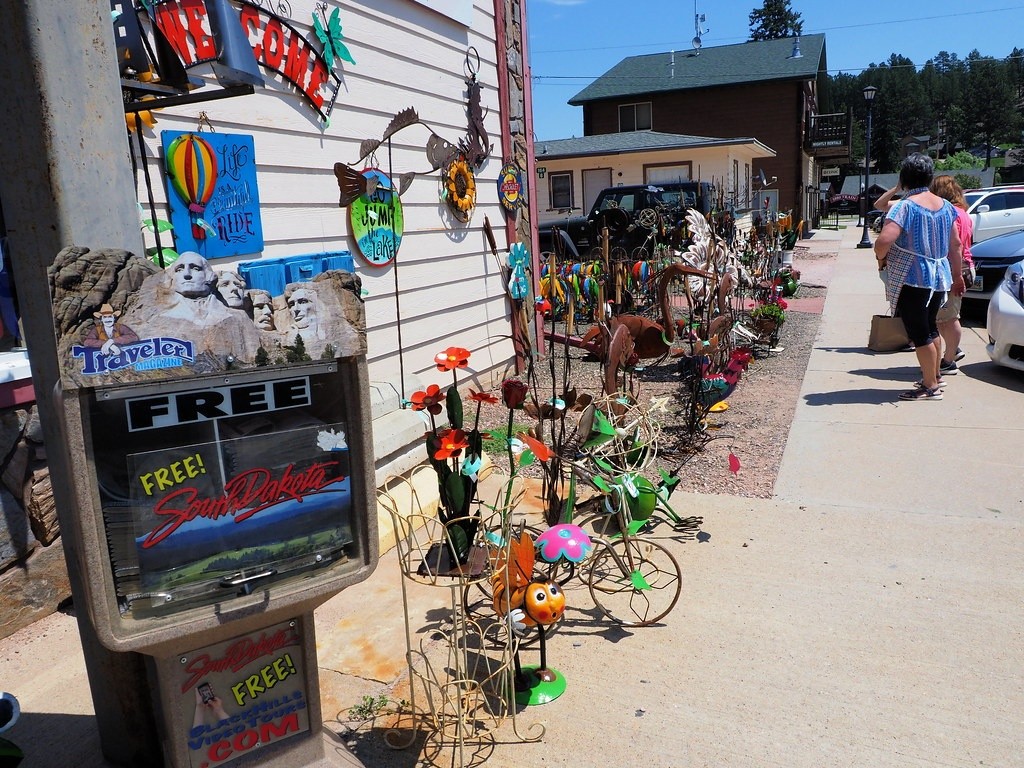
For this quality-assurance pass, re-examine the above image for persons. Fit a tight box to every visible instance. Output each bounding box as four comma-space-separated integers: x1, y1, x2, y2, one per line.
919, 175, 976, 374
873, 177, 915, 352
286, 283, 330, 338
873, 153, 968, 399
209, 269, 248, 313
160, 252, 232, 326
240, 287, 277, 330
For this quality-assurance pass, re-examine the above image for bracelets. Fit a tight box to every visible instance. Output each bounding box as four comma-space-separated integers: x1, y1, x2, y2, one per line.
875, 255, 888, 262
895, 184, 900, 191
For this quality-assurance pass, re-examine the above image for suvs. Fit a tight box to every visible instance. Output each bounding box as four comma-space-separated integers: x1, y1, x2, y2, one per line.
539, 182, 717, 260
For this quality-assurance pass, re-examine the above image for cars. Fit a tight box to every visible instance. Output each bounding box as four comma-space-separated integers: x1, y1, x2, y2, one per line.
867, 185, 1024, 371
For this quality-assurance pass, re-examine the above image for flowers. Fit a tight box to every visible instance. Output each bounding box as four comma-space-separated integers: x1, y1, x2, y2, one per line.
410, 345, 531, 565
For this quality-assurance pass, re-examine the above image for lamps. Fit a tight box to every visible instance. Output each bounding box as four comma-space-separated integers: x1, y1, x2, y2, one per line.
813, 187, 820, 194
805, 185, 815, 193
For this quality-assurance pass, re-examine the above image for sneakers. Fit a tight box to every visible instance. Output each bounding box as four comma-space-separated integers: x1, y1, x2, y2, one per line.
943, 347, 965, 362
920, 358, 958, 374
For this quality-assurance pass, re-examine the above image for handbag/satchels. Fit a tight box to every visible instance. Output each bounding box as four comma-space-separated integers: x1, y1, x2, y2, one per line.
867, 307, 910, 352
949, 258, 974, 292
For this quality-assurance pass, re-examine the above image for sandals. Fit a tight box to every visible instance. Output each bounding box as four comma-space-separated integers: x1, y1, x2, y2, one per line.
913, 375, 947, 387
898, 385, 943, 401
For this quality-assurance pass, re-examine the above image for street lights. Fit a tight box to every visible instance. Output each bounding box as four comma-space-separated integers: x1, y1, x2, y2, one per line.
857, 83, 877, 248
